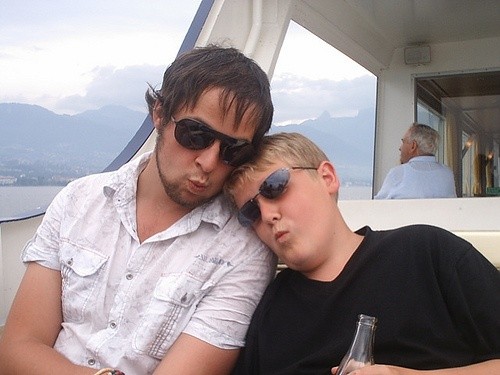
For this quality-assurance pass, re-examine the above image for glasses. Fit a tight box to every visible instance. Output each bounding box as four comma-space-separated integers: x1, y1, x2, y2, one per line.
237, 166, 326, 226
166, 111, 254, 166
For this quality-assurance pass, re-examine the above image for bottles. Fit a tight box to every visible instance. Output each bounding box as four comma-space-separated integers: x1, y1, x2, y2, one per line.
334, 313, 377, 375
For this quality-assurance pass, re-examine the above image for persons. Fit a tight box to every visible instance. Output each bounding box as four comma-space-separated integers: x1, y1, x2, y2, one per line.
0, 42, 279, 375
374, 122, 457, 199
223, 134, 500, 375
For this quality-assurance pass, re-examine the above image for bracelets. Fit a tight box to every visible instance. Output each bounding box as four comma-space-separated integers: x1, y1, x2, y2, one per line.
92, 368, 126, 375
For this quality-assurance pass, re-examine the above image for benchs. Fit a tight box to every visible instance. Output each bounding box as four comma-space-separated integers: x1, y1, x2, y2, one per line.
270, 199, 500, 271
